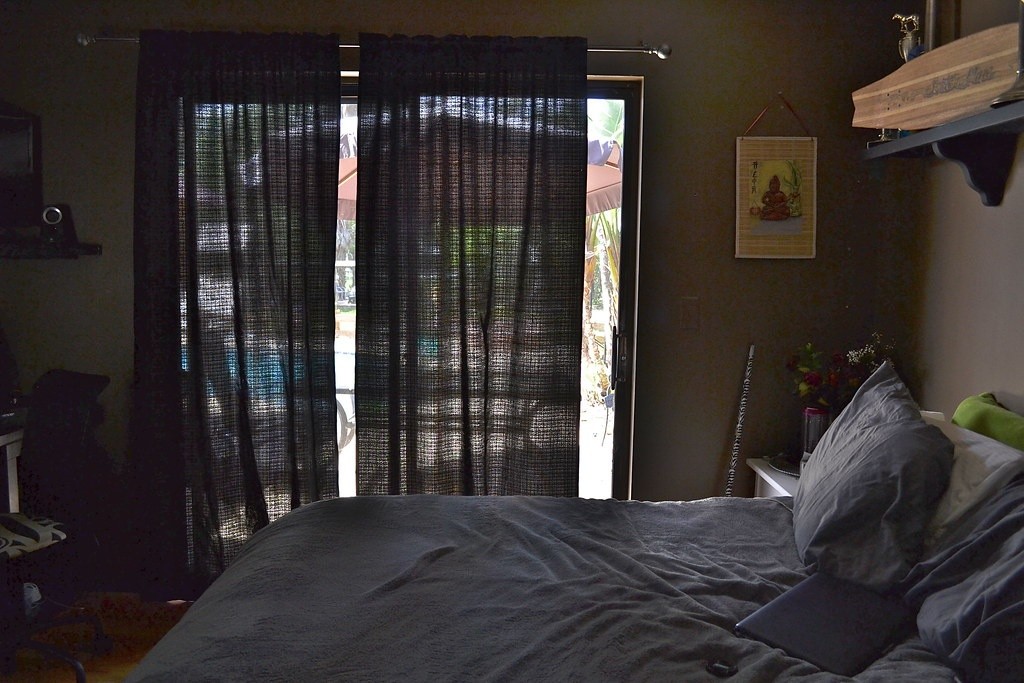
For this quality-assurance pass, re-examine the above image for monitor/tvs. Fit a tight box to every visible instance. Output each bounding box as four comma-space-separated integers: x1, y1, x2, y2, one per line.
0, 115, 42, 229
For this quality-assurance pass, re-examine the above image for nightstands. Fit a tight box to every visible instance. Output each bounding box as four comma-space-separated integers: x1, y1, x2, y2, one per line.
746, 457, 802, 500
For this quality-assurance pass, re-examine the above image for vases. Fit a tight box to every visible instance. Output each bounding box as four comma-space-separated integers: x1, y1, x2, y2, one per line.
801, 404, 835, 457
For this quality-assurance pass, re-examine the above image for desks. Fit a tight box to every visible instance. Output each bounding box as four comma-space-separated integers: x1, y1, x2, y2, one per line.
0, 404, 25, 512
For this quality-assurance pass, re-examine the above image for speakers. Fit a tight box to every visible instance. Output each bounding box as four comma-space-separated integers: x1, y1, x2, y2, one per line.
41, 204, 78, 244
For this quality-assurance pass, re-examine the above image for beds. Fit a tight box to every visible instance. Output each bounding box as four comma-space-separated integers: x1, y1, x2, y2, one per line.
130, 495, 1024, 683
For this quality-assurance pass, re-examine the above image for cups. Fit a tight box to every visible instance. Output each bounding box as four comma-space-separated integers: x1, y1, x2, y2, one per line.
801, 407, 827, 458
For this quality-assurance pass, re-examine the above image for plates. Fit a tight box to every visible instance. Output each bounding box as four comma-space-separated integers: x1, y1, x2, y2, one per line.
768, 455, 800, 476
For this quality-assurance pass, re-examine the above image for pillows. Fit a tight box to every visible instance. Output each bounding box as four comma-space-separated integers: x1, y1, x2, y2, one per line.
796, 362, 952, 566
921, 410, 1024, 565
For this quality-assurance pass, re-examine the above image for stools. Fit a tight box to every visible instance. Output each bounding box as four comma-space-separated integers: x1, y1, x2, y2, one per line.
0, 513, 111, 683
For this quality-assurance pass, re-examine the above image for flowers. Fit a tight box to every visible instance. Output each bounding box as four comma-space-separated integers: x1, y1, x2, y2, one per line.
785, 332, 895, 406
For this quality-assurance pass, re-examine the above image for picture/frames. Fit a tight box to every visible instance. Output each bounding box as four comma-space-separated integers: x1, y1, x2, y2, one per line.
735, 135, 818, 259
